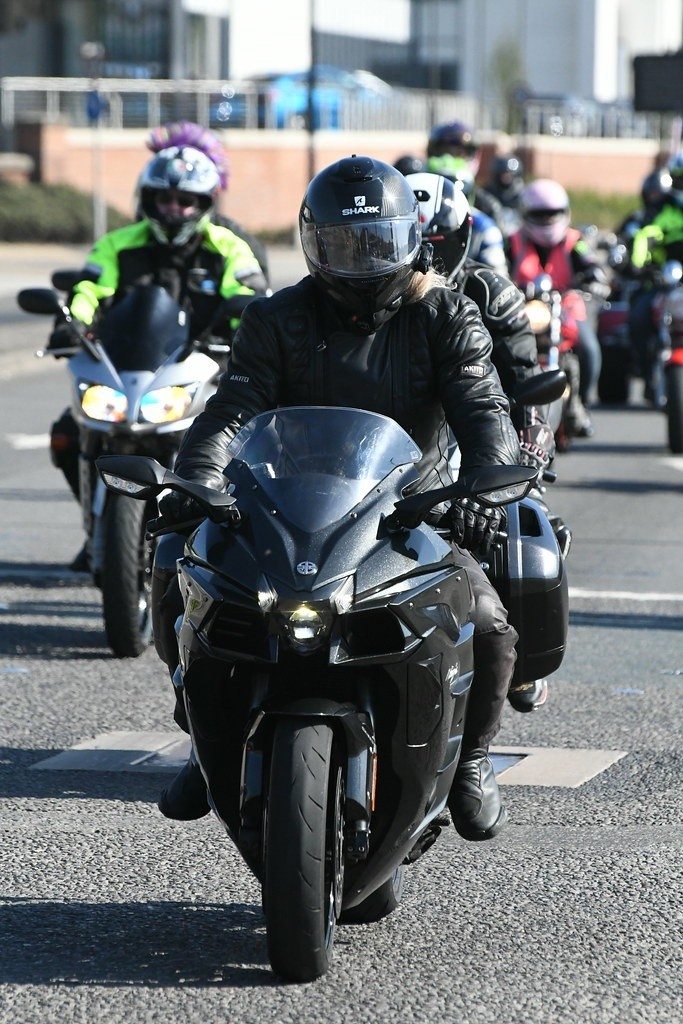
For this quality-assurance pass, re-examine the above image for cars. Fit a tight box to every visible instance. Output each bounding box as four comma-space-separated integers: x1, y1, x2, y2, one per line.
194, 64, 407, 132
521, 93, 616, 134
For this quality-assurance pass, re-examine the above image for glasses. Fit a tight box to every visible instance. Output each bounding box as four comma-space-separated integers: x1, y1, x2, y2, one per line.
154, 191, 198, 207
529, 210, 558, 217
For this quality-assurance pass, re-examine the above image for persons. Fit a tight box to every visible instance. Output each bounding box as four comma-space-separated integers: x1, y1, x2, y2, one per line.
151, 157, 525, 840
142, 118, 682, 459
52, 146, 274, 580
395, 170, 553, 713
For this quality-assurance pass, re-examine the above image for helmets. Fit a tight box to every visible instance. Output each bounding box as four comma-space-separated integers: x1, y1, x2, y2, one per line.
642, 172, 672, 213
402, 173, 474, 285
298, 155, 421, 331
136, 145, 219, 247
519, 178, 571, 247
146, 120, 229, 189
667, 154, 683, 206
426, 122, 481, 193
490, 155, 523, 199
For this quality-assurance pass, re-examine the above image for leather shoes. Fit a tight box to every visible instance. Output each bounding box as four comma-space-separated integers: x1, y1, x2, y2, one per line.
447, 740, 506, 841
157, 747, 212, 821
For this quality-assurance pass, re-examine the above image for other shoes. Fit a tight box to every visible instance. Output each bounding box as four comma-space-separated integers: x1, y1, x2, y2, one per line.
68, 543, 89, 571
577, 407, 592, 437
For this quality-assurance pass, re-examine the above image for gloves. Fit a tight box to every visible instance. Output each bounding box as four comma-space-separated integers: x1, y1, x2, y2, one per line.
159, 489, 207, 535
452, 497, 501, 557
519, 424, 555, 488
50, 321, 85, 359
583, 281, 611, 297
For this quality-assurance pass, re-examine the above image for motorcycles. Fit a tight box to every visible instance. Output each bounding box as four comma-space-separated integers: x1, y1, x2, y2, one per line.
88, 405, 546, 989
14, 285, 270, 659
441, 224, 681, 682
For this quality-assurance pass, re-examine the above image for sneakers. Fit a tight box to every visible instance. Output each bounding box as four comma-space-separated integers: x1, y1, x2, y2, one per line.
506, 677, 548, 713
